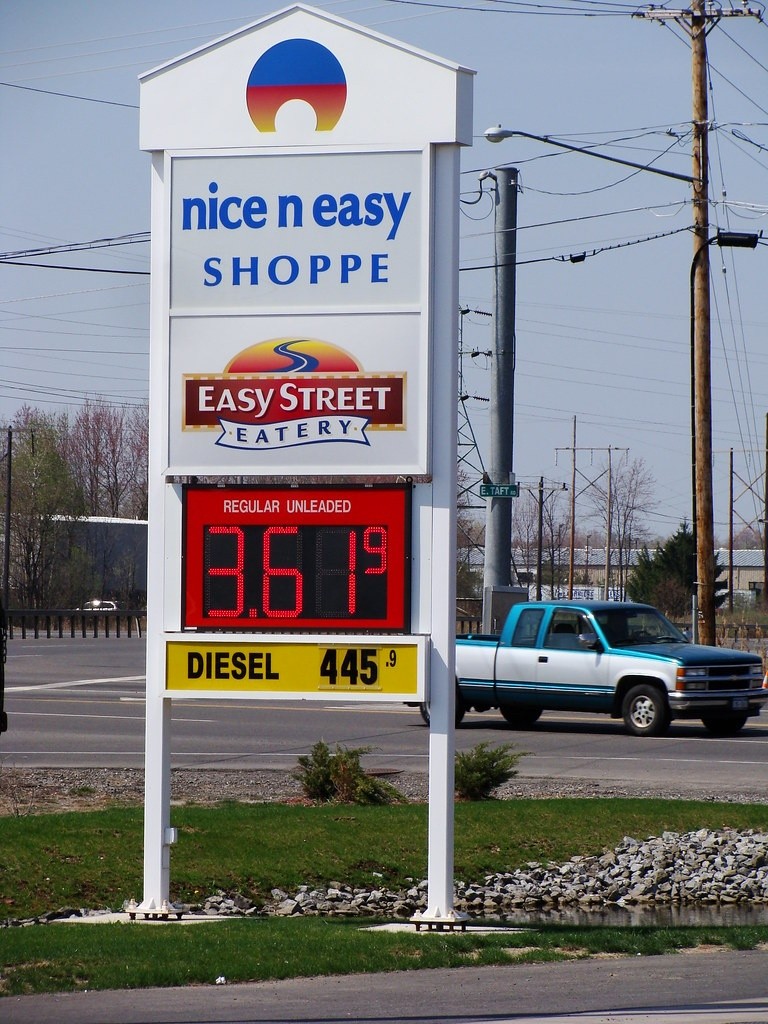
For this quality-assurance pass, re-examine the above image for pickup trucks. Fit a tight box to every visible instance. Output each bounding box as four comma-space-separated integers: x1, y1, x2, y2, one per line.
402, 599, 768, 737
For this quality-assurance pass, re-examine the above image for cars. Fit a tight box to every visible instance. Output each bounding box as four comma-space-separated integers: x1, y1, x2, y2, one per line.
76, 600, 130, 611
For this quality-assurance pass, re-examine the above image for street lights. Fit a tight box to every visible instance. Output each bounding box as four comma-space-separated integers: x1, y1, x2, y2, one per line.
558, 524, 564, 599
692, 232, 757, 645
585, 535, 592, 579
483, 124, 714, 645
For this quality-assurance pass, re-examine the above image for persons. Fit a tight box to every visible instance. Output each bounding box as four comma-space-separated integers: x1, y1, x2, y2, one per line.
682, 626, 693, 642
552, 624, 589, 650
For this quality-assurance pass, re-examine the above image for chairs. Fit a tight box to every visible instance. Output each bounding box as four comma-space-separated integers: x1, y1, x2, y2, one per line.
554, 623, 577, 648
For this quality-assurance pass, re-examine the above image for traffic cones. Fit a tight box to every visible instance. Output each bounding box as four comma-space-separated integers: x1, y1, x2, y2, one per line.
762, 672, 768, 688
